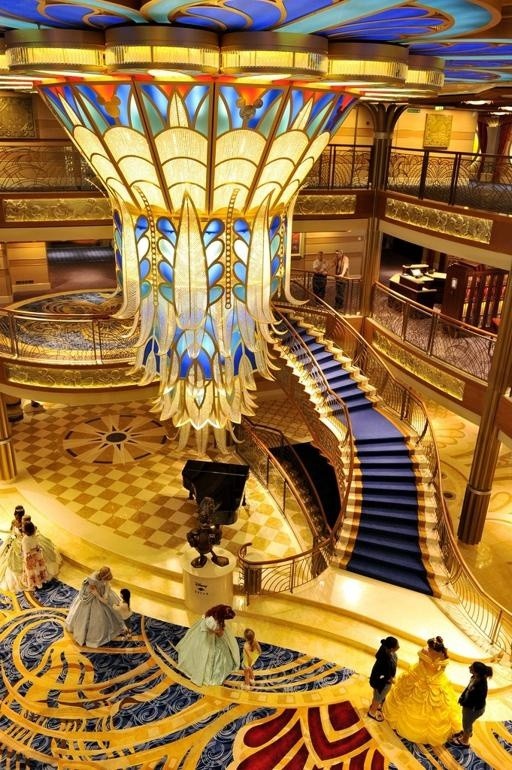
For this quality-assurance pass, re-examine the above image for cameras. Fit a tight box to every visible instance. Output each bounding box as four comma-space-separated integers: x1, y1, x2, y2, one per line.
335, 274, 339, 280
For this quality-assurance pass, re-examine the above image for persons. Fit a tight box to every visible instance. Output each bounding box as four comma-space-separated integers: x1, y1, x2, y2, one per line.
446, 660, 494, 749
8, 504, 39, 579
240, 628, 261, 689
410, 633, 451, 743
76, 564, 114, 647
195, 604, 236, 683
331, 249, 350, 310
311, 251, 328, 307
20, 521, 51, 590
22, 514, 37, 533
366, 636, 400, 723
110, 588, 135, 641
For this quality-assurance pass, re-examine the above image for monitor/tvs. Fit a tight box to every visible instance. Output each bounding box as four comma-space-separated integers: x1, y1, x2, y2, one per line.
411, 269, 423, 277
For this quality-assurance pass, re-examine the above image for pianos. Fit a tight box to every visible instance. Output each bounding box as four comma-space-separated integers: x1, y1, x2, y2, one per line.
181, 459, 250, 524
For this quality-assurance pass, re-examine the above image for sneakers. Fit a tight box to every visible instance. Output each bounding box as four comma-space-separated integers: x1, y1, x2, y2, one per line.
368, 704, 384, 722
241, 679, 255, 690
122, 629, 131, 640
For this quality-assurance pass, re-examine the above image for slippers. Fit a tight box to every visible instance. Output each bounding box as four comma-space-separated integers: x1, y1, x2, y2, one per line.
448, 730, 472, 748
